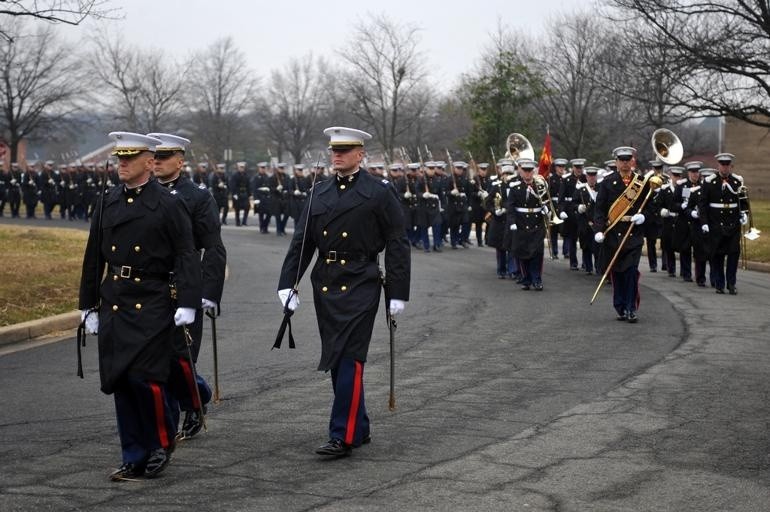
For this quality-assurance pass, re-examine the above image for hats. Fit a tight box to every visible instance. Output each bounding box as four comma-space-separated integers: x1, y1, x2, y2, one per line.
145, 131, 192, 158
552, 156, 617, 175
106, 131, 163, 158
321, 124, 374, 151
366, 157, 543, 176
649, 152, 741, 178
0, 160, 116, 168
182, 160, 325, 171
612, 146, 638, 160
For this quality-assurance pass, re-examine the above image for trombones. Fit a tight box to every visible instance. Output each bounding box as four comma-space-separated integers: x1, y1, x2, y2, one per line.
533, 174, 564, 261
736, 186, 761, 271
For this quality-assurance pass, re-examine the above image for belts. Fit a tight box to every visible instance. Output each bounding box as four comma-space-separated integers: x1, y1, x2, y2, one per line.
315, 248, 380, 266
709, 202, 738, 209
514, 206, 543, 214
105, 262, 176, 282
615, 214, 635, 222
551, 197, 574, 203
669, 211, 681, 217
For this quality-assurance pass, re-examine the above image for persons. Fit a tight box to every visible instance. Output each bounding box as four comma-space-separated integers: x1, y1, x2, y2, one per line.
0, 160, 121, 223
276, 126, 411, 457
364, 147, 750, 324
146, 132, 227, 440
182, 160, 327, 237
78, 130, 202, 482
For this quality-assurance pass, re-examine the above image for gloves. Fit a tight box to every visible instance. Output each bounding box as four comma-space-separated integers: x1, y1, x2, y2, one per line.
660, 207, 670, 218
402, 189, 489, 200
81, 306, 99, 335
630, 213, 646, 225
577, 204, 587, 214
739, 214, 748, 225
172, 306, 197, 327
387, 298, 405, 315
200, 298, 217, 311
276, 288, 301, 311
509, 223, 518, 231
690, 210, 699, 219
701, 224, 710, 234
558, 210, 568, 220
593, 231, 606, 244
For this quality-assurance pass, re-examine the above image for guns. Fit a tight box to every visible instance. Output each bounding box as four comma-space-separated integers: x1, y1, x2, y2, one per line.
288, 153, 299, 190
267, 149, 283, 193
204, 153, 222, 182
382, 144, 499, 192
304, 151, 315, 185
190, 151, 205, 183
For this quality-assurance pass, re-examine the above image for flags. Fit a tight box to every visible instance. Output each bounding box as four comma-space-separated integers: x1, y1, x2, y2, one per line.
537, 133, 551, 179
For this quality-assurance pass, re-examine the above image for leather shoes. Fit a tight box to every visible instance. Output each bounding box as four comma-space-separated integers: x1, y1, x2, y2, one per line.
727, 282, 738, 296
351, 429, 374, 449
314, 435, 354, 457
616, 309, 627, 323
411, 238, 491, 252
108, 454, 142, 481
142, 440, 177, 479
549, 253, 612, 283
715, 288, 725, 294
649, 263, 714, 287
627, 310, 639, 323
496, 270, 544, 291
221, 222, 299, 236
177, 403, 208, 443
